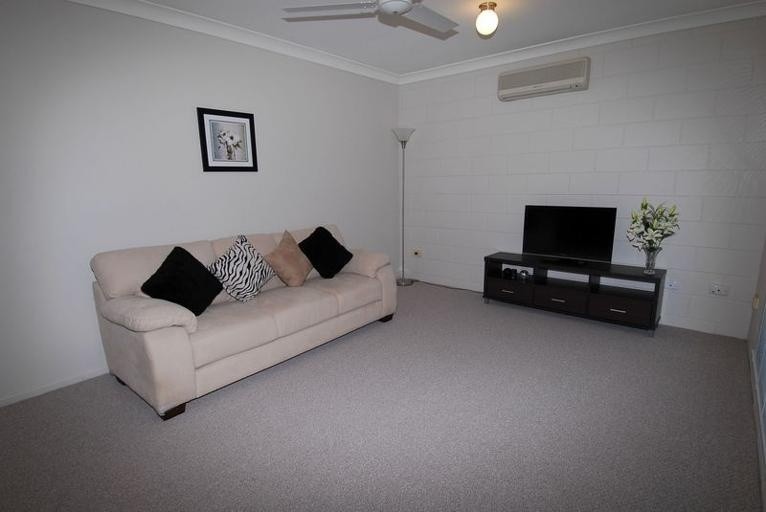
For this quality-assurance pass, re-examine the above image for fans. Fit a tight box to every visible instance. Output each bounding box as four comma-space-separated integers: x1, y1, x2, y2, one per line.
280, 0, 459, 34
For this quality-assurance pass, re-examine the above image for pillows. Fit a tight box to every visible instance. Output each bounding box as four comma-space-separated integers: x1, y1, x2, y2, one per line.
140, 246, 225, 316
298, 226, 354, 281
207, 233, 276, 302
264, 230, 314, 287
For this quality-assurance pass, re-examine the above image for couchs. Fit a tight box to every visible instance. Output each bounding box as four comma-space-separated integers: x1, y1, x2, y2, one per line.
89, 225, 397, 422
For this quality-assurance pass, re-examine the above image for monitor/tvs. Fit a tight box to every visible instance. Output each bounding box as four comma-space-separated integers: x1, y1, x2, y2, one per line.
521, 204, 618, 271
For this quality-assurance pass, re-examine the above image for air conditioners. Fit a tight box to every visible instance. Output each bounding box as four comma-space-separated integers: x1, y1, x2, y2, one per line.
497, 56, 590, 102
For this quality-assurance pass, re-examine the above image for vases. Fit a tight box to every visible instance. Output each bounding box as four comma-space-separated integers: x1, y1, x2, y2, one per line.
641, 246, 662, 274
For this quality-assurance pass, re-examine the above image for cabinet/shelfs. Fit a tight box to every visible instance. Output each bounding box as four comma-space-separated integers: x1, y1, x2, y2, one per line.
482, 251, 667, 331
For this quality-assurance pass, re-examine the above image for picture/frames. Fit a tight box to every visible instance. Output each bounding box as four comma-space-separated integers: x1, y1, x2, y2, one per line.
196, 106, 258, 172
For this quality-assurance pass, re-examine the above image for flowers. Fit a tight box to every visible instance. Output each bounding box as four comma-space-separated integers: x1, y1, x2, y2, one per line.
626, 199, 681, 252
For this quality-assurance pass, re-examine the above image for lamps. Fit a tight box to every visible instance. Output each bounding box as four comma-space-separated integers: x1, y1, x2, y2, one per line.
475, 2, 499, 37
392, 128, 416, 286
